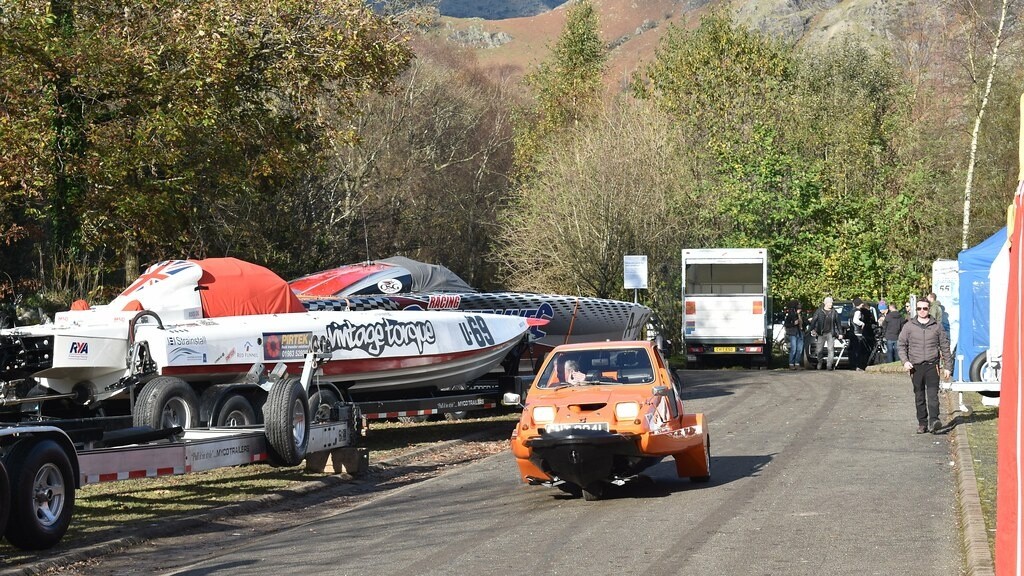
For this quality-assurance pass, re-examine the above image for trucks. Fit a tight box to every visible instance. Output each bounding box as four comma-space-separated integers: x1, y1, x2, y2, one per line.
681, 248, 773, 371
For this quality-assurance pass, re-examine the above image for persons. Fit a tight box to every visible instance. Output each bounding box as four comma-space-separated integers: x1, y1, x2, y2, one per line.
554, 355, 579, 382
850, 298, 902, 371
898, 298, 952, 433
784, 304, 809, 370
928, 293, 943, 322
810, 297, 843, 370
905, 301, 910, 312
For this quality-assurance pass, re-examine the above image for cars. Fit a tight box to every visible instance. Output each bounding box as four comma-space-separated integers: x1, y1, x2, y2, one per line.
774, 299, 883, 366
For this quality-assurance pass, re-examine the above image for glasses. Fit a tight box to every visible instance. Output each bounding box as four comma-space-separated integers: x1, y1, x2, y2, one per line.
917, 307, 928, 311
906, 306, 909, 307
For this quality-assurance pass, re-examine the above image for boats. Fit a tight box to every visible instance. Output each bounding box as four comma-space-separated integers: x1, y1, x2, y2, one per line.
2, 253, 653, 405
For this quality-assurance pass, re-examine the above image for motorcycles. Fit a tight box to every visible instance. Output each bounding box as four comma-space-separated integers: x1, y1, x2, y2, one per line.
503, 340, 711, 501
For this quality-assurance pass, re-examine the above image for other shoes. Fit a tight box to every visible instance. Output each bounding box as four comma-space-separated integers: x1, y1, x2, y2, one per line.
816, 362, 823, 369
929, 418, 942, 433
827, 363, 833, 370
795, 365, 802, 370
917, 425, 928, 434
789, 364, 796, 370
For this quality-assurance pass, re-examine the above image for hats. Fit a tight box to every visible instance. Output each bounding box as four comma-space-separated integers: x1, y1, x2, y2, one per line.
796, 305, 802, 309
878, 301, 888, 310
854, 298, 862, 306
